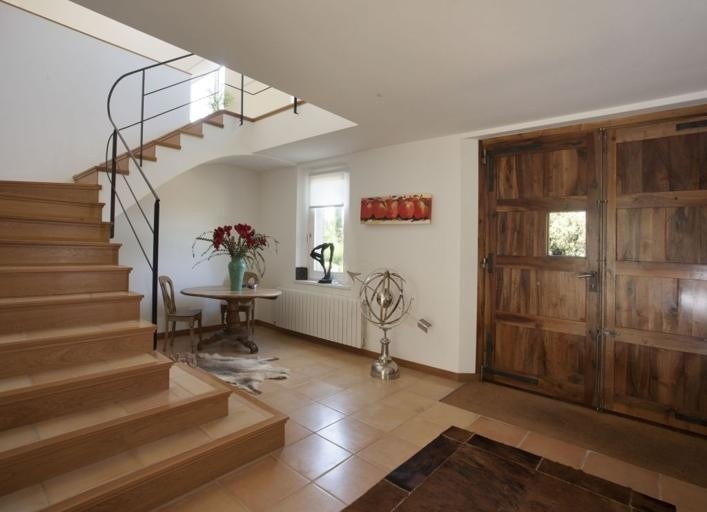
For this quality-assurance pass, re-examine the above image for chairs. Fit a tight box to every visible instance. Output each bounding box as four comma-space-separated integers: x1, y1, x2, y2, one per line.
159, 275, 202, 352
219, 273, 257, 332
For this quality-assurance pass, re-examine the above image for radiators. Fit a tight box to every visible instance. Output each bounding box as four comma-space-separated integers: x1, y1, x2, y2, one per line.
272, 286, 367, 350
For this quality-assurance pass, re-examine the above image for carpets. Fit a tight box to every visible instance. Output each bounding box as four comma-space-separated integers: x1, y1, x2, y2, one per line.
341, 426, 677, 511
439, 380, 706, 488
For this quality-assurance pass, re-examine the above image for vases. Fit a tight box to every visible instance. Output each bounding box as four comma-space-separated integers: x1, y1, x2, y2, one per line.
228, 255, 245, 290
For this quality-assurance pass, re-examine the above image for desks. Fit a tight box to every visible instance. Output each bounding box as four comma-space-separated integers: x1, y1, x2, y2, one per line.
177, 286, 283, 353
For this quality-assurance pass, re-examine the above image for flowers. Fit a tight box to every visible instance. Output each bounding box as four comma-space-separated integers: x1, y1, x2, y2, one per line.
188, 223, 280, 277
